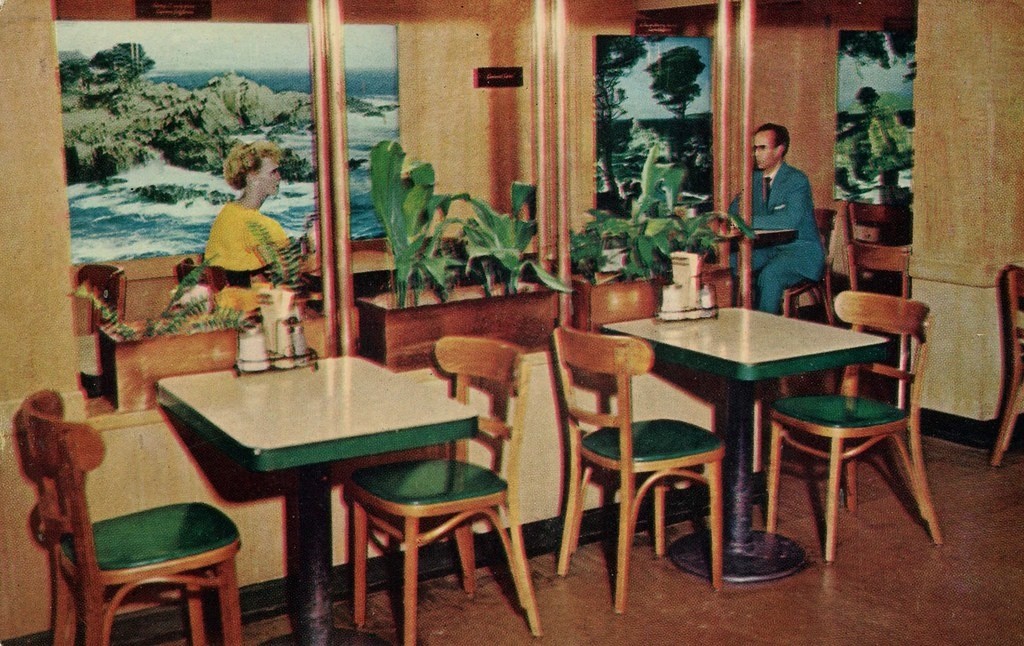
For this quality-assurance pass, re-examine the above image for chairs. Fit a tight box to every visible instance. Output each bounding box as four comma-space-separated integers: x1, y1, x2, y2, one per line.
551, 323, 727, 616
986, 264, 1024, 468
841, 200, 901, 297
766, 289, 945, 562
16, 390, 244, 646
344, 336, 544, 646
846, 238, 910, 410
783, 208, 837, 325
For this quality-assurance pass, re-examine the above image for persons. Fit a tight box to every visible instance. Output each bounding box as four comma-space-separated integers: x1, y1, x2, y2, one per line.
725, 122, 828, 318
203, 138, 319, 329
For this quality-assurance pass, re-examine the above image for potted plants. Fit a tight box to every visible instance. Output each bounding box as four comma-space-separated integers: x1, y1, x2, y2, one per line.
357, 140, 579, 371
58, 206, 319, 409
541, 146, 735, 334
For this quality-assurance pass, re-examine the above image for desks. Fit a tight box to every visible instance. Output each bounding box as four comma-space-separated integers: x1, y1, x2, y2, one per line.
603, 305, 891, 582
156, 352, 480, 646
